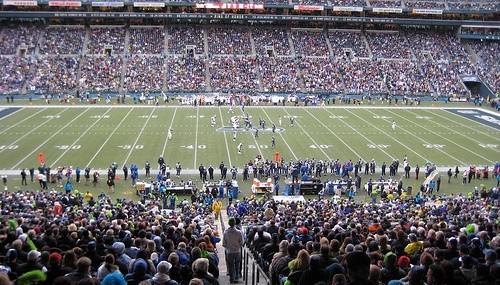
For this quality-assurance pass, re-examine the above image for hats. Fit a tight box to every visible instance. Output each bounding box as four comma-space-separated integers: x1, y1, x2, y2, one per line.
133, 258, 148, 270
228, 217, 236, 225
157, 261, 172, 273
28, 250, 41, 260
0, 191, 335, 285
49, 252, 61, 261
77, 257, 92, 266
398, 256, 410, 267
88, 241, 96, 248
6, 248, 17, 256
383, 252, 397, 264
459, 255, 473, 262
447, 237, 457, 245
483, 249, 497, 256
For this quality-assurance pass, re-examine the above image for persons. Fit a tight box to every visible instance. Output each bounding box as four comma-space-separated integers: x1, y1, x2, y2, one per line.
392, 120, 396, 131
0, 0, 500, 111
212, 113, 294, 155
1, 152, 500, 285
168, 126, 173, 140
